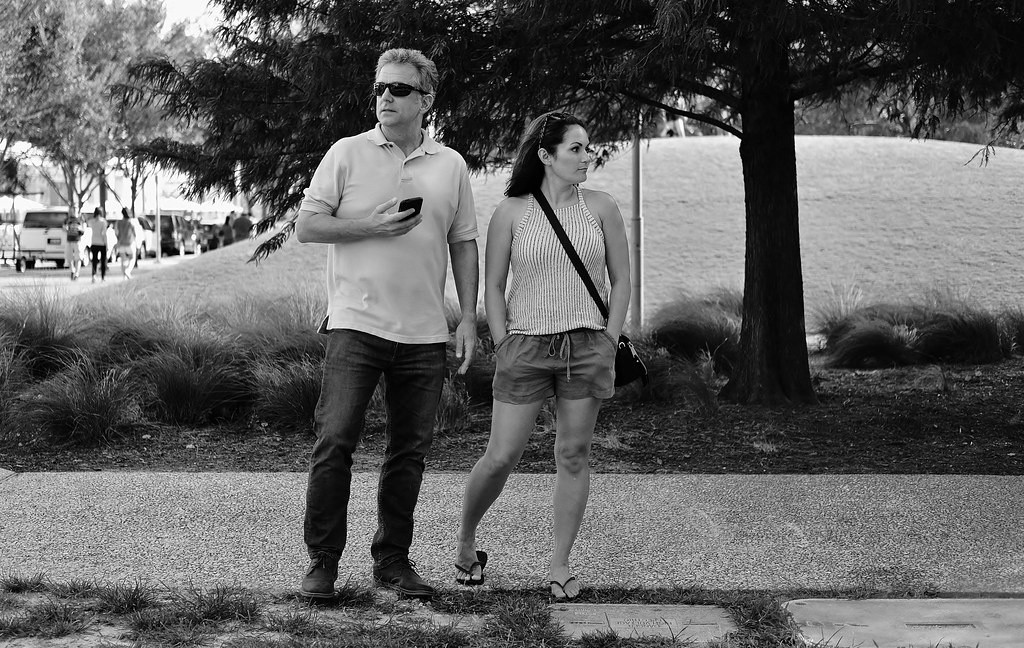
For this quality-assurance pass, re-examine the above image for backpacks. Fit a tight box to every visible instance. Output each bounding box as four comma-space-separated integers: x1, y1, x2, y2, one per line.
66, 220, 80, 242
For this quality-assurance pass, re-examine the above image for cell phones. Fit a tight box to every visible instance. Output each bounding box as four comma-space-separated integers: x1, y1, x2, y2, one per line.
398, 197, 423, 222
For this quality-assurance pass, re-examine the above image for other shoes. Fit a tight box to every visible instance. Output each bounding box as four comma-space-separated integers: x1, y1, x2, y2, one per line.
74, 273, 79, 280
70, 269, 75, 281
124, 269, 132, 280
91, 277, 96, 284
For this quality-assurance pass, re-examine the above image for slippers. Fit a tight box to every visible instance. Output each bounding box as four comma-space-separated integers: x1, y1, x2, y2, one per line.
456, 550, 487, 585
549, 577, 580, 602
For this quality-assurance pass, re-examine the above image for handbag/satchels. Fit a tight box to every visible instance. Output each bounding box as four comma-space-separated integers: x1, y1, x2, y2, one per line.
614, 333, 647, 387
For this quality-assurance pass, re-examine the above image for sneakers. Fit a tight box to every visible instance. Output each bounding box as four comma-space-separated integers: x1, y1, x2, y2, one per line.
373, 559, 433, 596
299, 551, 339, 597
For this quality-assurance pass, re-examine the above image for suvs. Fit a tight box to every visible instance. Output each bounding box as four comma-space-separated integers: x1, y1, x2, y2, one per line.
107, 216, 157, 259
15, 206, 92, 273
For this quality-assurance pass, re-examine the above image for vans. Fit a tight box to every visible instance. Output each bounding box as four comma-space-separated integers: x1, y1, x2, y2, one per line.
146, 215, 196, 256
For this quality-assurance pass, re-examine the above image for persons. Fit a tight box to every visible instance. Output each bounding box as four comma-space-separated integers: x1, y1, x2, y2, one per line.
296, 49, 478, 598
997, 135, 1016, 147
62, 206, 139, 283
453, 109, 629, 603
207, 210, 276, 252
655, 88, 688, 138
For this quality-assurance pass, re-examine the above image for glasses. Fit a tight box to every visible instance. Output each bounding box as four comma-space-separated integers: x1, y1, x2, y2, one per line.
372, 81, 430, 97
539, 111, 574, 150
